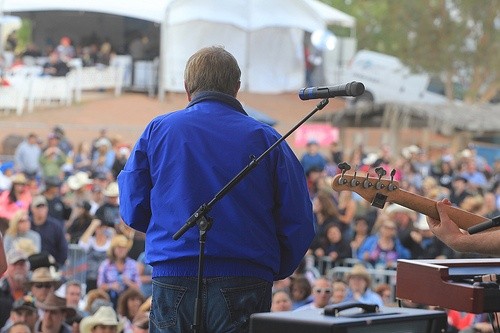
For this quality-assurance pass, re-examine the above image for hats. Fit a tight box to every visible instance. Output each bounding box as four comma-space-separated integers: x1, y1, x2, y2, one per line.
79, 306, 126, 333
344, 264, 371, 287
94, 138, 112, 150
67, 171, 94, 191
32, 194, 48, 207
37, 294, 76, 318
107, 236, 133, 258
12, 295, 39, 312
103, 181, 120, 197
7, 249, 28, 264
11, 173, 29, 185
28, 252, 59, 273
30, 267, 60, 282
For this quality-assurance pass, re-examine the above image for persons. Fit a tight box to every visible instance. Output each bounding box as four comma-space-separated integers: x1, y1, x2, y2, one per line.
426, 196, 500, 257
0, 26, 160, 94
117, 45, 316, 332
0, 126, 155, 333
268, 139, 499, 333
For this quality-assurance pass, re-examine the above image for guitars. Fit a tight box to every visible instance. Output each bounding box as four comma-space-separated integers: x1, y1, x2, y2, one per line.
331, 162, 500, 233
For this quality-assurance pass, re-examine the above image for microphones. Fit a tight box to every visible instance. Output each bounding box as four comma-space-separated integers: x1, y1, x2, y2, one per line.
299, 80, 365, 100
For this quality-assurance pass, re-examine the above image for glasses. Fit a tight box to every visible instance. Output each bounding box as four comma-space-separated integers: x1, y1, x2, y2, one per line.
32, 283, 53, 288
136, 322, 149, 330
316, 288, 331, 294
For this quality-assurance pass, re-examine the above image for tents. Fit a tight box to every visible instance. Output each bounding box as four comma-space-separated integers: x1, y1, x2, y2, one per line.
0, 0, 357, 95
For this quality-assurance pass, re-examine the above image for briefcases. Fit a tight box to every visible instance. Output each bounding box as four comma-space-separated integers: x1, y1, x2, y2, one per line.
249, 301, 447, 333
